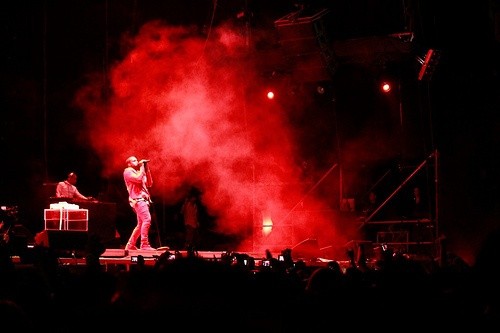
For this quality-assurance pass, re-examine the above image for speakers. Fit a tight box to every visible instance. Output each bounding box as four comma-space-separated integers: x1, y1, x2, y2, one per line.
291, 238, 320, 261
33, 229, 101, 258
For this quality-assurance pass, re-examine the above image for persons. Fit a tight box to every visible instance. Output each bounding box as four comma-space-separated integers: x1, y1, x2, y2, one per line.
408, 185, 425, 219
362, 192, 383, 222
0, 243, 500, 333
122, 157, 159, 253
55, 172, 92, 204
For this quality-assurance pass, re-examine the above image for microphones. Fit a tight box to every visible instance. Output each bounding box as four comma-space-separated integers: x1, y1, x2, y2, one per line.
143, 160, 150, 163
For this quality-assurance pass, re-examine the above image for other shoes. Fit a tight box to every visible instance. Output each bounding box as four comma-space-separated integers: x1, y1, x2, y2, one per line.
139, 245, 157, 251
124, 243, 139, 251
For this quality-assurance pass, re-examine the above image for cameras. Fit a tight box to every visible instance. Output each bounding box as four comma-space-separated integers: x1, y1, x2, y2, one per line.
262, 261, 270, 267
131, 256, 138, 262
279, 255, 284, 262
167, 255, 175, 260
